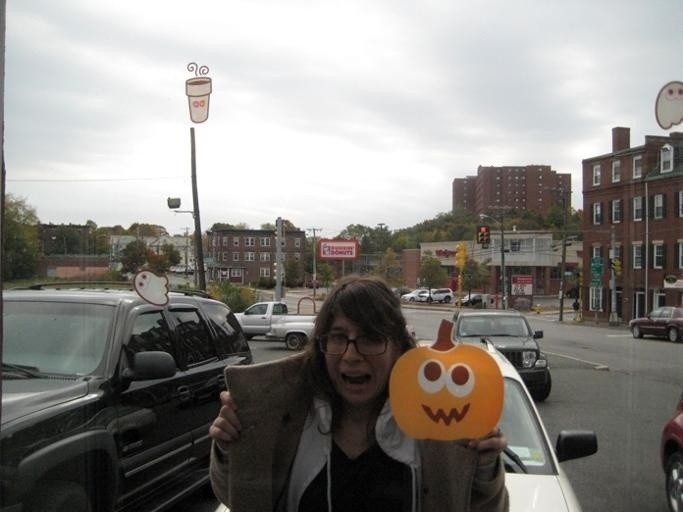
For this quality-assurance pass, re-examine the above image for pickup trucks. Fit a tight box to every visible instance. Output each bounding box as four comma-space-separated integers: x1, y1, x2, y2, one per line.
233, 301, 318, 340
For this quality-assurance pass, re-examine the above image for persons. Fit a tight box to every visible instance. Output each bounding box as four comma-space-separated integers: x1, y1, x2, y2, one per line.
572, 298, 580, 321
207, 270, 513, 512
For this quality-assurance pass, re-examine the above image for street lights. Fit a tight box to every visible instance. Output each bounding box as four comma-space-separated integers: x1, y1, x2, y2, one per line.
306, 228, 322, 298
480, 214, 505, 309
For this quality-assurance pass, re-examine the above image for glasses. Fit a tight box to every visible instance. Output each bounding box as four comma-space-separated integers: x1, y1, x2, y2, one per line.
318, 330, 393, 358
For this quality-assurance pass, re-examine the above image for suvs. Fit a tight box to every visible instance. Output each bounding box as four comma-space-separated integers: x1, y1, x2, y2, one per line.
1, 286, 252, 510
451, 309, 550, 401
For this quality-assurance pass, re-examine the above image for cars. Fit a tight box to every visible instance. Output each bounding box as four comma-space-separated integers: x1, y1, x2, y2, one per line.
454, 294, 493, 306
415, 337, 597, 512
659, 394, 682, 512
628, 306, 682, 343
390, 287, 452, 304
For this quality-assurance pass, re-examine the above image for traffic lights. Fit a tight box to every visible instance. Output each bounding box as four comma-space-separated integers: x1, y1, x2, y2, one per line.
166, 198, 181, 208
476, 225, 491, 249
